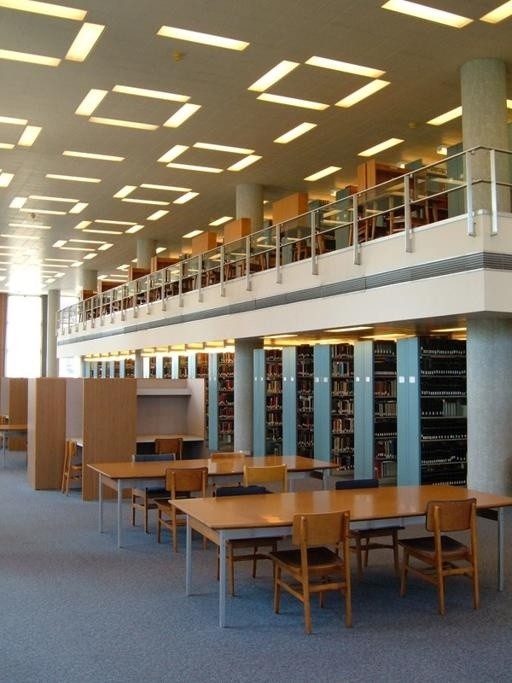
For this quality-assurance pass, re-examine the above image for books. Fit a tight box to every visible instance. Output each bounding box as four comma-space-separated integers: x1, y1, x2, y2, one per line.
217, 353, 234, 441
331, 342, 355, 471
89, 356, 188, 378
373, 340, 397, 477
198, 353, 209, 428
296, 346, 315, 459
421, 339, 466, 485
266, 350, 283, 456
